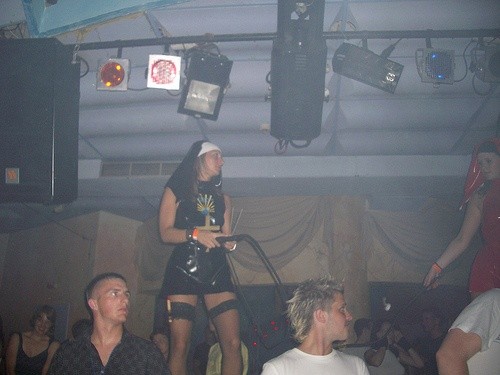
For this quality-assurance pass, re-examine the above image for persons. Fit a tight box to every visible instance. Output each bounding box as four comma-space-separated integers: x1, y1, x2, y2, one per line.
422, 137, 500, 298
7, 305, 59, 375
343, 307, 448, 375
157, 140, 242, 375
150, 327, 170, 362
434, 288, 500, 375
72, 318, 91, 339
206, 315, 249, 375
260, 274, 370, 375
193, 326, 217, 375
46, 273, 172, 375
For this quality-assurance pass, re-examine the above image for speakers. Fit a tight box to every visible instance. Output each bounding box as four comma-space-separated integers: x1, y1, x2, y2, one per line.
0, 39, 79, 205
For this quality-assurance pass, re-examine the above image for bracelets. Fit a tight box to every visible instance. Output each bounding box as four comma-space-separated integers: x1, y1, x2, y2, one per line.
431, 262, 442, 273
229, 242, 237, 251
185, 226, 199, 243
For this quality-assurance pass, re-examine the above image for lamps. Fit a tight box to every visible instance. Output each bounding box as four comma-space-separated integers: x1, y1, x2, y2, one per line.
178, 50, 234, 122
415, 47, 455, 87
269, 0, 326, 155
332, 42, 405, 95
146, 54, 181, 91
96, 58, 130, 93
468, 44, 500, 82
